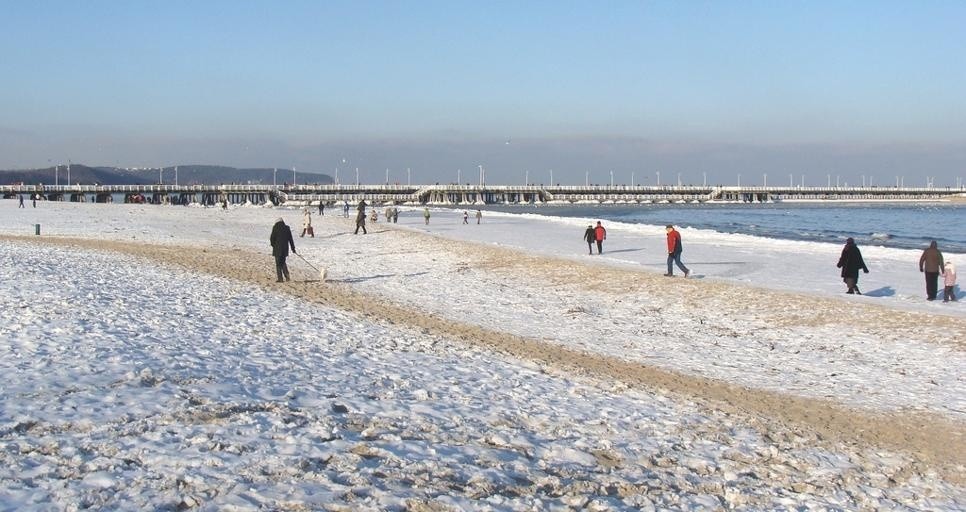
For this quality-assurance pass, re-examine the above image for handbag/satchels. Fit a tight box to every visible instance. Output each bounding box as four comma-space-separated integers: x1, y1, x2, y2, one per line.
306, 226, 313, 235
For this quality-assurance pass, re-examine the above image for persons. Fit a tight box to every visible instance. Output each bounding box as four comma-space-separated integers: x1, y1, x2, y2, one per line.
920, 241, 944, 300
463, 211, 469, 225
18, 194, 25, 208
270, 217, 295, 282
300, 208, 314, 238
594, 221, 606, 254
476, 210, 482, 224
664, 225, 690, 278
939, 262, 956, 302
837, 237, 868, 295
583, 225, 594, 254
424, 208, 430, 225
318, 199, 399, 234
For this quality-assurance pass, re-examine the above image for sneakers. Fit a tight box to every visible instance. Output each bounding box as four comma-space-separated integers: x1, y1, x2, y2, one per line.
663, 272, 674, 277
684, 270, 691, 278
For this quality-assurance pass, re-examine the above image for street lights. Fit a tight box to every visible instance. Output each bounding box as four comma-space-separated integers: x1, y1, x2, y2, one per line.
158, 166, 163, 184
292, 166, 296, 186
65, 158, 70, 186
54, 165, 59, 185
273, 167, 276, 185
173, 162, 178, 186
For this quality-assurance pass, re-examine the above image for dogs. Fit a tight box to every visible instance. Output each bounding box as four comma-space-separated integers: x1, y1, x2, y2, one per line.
320, 268, 327, 280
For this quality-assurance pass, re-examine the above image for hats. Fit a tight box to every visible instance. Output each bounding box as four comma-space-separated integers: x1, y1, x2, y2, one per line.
273, 216, 283, 224
664, 224, 674, 229
